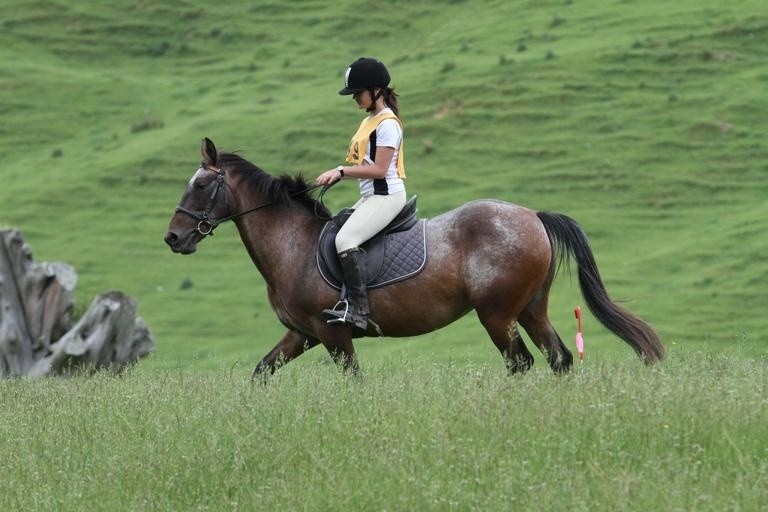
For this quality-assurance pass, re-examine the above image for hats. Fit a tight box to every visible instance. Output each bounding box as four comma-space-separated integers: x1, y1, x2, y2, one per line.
337, 58, 390, 95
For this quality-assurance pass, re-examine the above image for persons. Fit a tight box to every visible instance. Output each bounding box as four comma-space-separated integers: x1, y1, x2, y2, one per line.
317, 57, 407, 331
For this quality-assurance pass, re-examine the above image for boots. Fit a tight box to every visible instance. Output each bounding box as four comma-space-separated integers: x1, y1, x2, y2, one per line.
321, 247, 371, 331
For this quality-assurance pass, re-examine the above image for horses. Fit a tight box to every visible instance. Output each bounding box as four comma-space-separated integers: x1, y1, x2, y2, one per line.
164, 136, 668, 388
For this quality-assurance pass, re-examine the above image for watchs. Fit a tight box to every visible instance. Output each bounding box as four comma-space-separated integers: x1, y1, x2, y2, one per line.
337, 165, 344, 177
